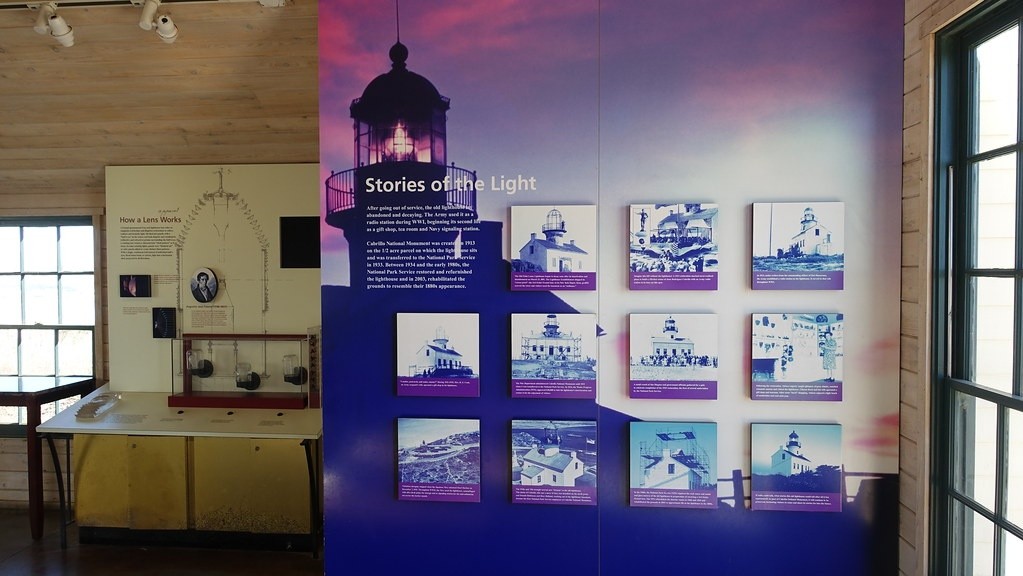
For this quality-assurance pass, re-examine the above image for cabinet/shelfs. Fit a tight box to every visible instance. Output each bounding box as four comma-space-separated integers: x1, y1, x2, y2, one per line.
72, 434, 323, 552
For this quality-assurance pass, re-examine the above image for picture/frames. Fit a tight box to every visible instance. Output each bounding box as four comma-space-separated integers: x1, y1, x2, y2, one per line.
191, 267, 217, 303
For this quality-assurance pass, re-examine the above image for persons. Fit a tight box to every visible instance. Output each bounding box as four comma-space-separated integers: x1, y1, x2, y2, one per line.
634, 209, 648, 231
630, 234, 711, 272
192, 272, 213, 302
